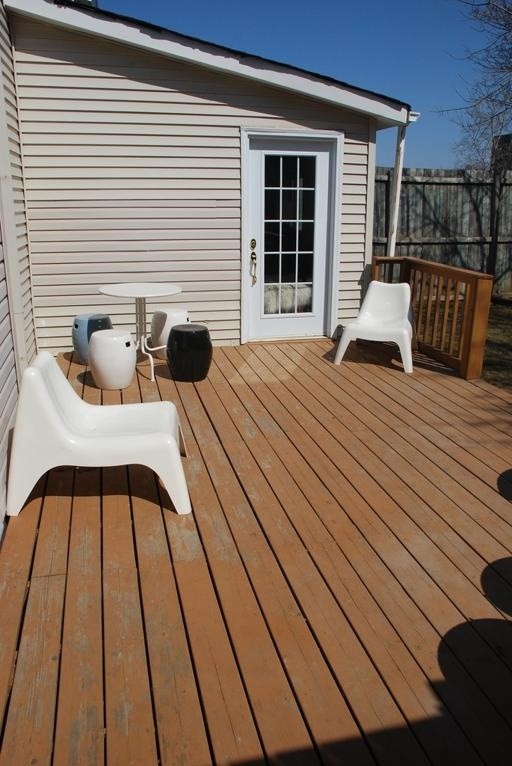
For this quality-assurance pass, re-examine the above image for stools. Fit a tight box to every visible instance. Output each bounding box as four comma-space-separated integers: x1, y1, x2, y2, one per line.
72, 283, 213, 391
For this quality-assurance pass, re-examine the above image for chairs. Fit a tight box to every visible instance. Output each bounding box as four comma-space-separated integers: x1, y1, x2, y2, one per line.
333, 280, 414, 374
7, 350, 193, 516
